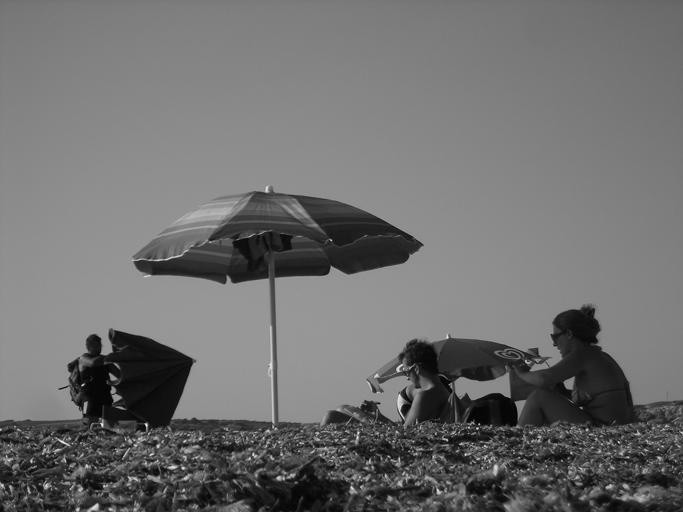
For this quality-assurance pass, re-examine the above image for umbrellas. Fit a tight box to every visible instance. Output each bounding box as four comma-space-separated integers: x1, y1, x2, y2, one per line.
58, 328, 195, 429
363, 332, 552, 423
130, 185, 425, 430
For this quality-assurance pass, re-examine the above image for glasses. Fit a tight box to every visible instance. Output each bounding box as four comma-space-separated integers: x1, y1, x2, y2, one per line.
551, 331, 568, 343
403, 363, 421, 377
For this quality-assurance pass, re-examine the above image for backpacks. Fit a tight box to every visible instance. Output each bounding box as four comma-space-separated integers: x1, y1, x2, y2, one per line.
68, 363, 87, 411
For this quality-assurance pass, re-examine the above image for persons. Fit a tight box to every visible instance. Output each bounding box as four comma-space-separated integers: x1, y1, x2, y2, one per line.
507, 304, 635, 427
320, 338, 451, 428
68, 335, 122, 422
